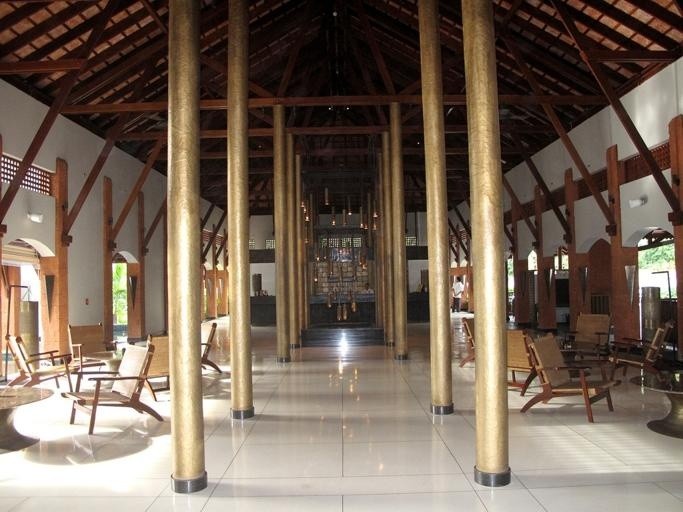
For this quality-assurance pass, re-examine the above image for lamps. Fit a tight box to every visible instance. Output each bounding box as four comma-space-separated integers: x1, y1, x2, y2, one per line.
301, 181, 379, 232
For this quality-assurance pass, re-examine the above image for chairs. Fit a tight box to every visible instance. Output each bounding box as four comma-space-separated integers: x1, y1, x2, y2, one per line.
5, 324, 223, 433
460, 310, 675, 421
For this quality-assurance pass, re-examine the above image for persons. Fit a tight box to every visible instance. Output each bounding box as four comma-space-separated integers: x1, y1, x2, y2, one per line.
452, 276, 464, 312
361, 283, 374, 294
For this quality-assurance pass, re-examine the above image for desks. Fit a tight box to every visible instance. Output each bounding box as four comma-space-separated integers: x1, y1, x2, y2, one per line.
0, 387, 53, 454
630, 370, 683, 439
